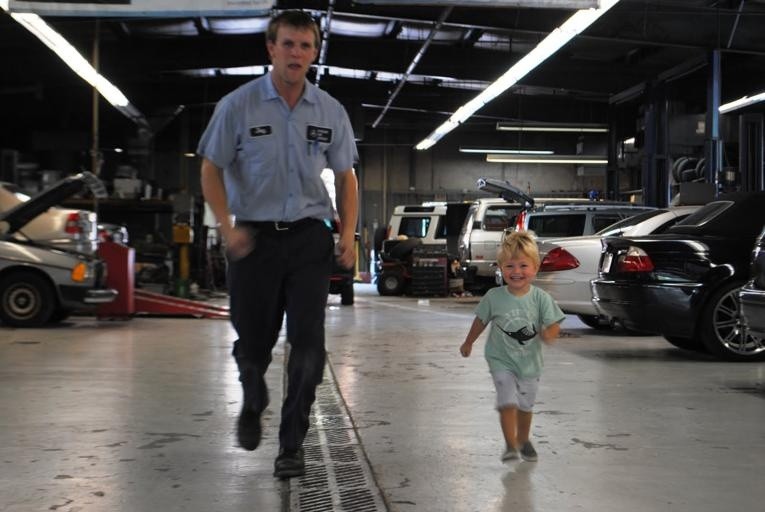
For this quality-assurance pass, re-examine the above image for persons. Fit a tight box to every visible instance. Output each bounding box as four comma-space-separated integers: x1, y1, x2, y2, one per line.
194, 10, 362, 481
459, 229, 565, 462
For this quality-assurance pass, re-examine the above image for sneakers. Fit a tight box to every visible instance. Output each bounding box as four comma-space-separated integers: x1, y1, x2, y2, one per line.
517, 437, 539, 462
501, 447, 520, 462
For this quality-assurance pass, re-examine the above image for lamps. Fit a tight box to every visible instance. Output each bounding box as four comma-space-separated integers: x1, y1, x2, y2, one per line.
0, 0, 152, 134
409, 0, 626, 170
718, 89, 765, 116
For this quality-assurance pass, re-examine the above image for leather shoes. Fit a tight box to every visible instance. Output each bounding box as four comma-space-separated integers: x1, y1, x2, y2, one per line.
272, 442, 307, 478
237, 403, 266, 451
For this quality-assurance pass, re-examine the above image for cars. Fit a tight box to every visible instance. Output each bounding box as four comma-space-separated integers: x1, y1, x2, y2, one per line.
1, 169, 132, 325
327, 214, 362, 306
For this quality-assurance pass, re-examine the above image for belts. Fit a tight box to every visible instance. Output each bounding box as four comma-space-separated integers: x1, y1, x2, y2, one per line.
232, 220, 335, 230
